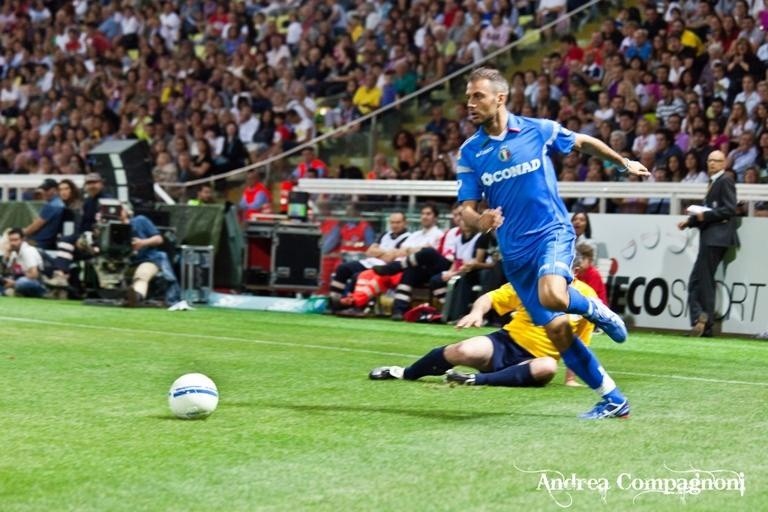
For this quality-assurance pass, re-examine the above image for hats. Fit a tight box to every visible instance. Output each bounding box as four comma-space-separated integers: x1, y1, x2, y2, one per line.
85, 173, 101, 183
38, 179, 58, 189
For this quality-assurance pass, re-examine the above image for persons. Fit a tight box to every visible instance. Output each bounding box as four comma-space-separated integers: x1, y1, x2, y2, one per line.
0, 0, 768, 337
369, 237, 599, 387
455, 68, 651, 419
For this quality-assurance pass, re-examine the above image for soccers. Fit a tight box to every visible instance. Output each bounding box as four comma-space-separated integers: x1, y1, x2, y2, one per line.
168, 373, 219, 419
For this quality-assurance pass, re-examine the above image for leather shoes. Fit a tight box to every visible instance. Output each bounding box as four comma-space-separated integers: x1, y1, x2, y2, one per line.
689, 313, 722, 336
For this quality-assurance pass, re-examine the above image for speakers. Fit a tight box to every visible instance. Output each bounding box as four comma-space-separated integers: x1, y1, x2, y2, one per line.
86, 139, 156, 201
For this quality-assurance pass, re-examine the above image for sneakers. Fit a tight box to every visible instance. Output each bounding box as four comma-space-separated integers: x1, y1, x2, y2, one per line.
43, 274, 68, 299
128, 285, 140, 307
587, 298, 627, 343
391, 310, 402, 320
374, 265, 398, 277
369, 366, 405, 380
579, 396, 630, 420
329, 297, 374, 316
445, 370, 476, 387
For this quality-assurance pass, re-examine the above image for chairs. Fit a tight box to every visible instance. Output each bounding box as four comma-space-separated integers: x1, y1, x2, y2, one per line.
596, 259, 612, 285
435, 282, 483, 320
411, 287, 433, 309
